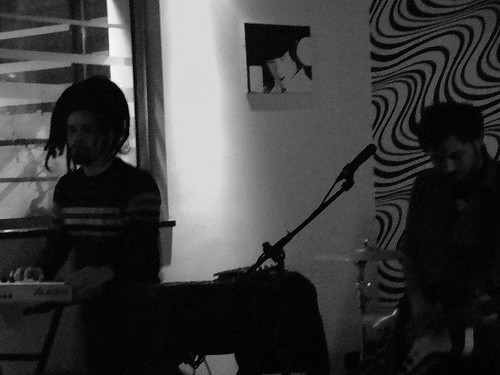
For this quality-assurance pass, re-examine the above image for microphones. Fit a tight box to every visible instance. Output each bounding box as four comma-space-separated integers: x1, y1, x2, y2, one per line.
338, 144, 380, 182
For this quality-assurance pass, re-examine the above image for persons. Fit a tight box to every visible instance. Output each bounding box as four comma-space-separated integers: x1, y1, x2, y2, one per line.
388, 100, 500, 375
9, 77, 162, 374
245, 23, 312, 94
